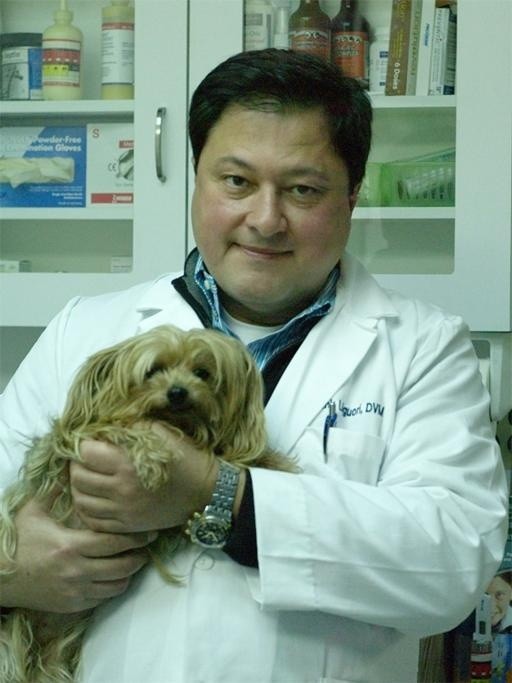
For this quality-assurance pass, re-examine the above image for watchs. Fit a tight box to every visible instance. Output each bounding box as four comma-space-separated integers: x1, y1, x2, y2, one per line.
184, 460, 241, 548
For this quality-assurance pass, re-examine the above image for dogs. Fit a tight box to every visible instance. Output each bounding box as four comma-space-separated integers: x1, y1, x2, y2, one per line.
0, 326, 303, 683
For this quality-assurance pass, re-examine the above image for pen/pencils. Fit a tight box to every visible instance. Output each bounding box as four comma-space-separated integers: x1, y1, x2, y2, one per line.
322, 402, 338, 464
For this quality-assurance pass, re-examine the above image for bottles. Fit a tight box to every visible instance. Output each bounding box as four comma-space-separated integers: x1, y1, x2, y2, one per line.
331, 0, 371, 92
100, 0, 136, 100
368, 25, 391, 96
288, 0, 332, 67
42, 0, 84, 100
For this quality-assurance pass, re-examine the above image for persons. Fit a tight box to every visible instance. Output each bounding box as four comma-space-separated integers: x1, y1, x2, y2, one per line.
0, 48, 509, 683
485, 571, 512, 634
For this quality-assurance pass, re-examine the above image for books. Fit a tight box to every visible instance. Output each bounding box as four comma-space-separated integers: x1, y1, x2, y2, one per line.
384, 0, 457, 96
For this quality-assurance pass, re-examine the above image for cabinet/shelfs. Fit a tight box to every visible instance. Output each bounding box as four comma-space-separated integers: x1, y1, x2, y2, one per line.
1, 1, 512, 333
